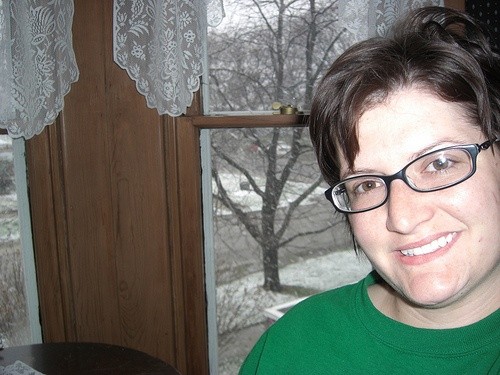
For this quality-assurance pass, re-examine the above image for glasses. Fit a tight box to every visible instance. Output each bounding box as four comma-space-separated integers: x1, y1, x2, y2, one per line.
324, 137, 498, 215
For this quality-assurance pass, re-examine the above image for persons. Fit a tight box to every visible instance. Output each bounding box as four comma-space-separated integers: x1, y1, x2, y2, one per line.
237, 5, 500, 375
